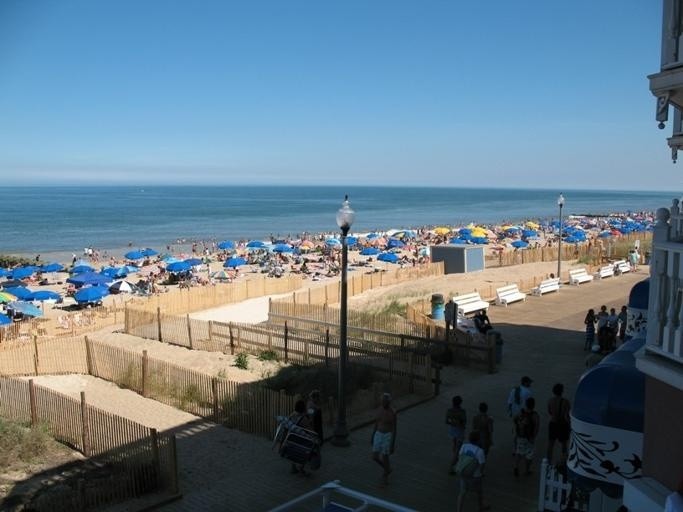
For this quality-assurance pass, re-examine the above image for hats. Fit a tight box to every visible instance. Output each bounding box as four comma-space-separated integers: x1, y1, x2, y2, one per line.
520, 376, 534, 384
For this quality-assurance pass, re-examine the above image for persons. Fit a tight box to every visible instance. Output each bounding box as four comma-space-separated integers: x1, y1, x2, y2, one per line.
445, 396, 467, 459
457, 430, 486, 511
512, 397, 540, 476
583, 305, 638, 349
307, 391, 323, 470
472, 402, 494, 458
288, 401, 310, 475
370, 393, 397, 472
508, 376, 534, 425
444, 296, 494, 336
547, 383, 571, 464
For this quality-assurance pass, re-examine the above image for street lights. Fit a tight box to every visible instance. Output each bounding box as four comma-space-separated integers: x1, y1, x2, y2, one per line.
555, 191, 564, 286
329, 194, 355, 446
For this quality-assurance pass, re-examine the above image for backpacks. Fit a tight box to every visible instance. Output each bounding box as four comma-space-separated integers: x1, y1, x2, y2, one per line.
455, 443, 480, 476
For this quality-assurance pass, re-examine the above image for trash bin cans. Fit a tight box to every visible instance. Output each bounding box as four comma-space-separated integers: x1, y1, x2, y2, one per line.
431, 294, 445, 320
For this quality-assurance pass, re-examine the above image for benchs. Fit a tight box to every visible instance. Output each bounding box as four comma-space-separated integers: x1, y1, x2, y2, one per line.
452, 259, 631, 317
